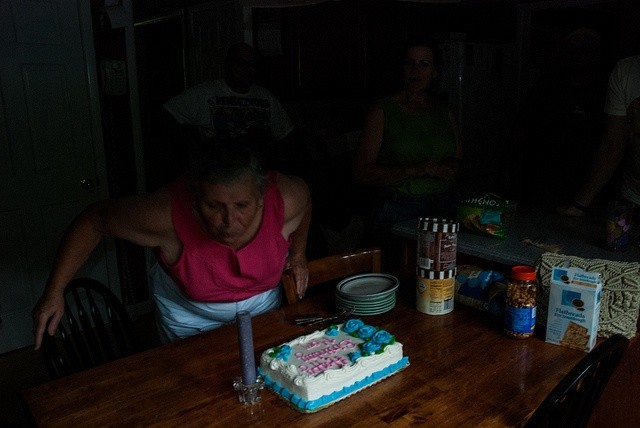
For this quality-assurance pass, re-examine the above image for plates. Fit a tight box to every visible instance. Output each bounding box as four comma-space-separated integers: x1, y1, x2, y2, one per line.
336, 271, 400, 317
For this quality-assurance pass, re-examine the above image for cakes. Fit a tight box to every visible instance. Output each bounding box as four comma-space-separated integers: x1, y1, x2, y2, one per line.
255, 320, 409, 411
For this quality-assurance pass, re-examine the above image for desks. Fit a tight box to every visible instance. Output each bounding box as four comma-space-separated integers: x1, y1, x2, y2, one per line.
392, 208, 640, 266
0, 269, 640, 428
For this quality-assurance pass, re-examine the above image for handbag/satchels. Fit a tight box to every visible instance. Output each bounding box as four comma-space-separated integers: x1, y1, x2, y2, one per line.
539, 250, 637, 346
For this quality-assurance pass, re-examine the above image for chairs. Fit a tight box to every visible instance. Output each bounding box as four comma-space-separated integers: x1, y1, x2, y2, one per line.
283, 249, 385, 304
526, 335, 627, 427
36, 277, 143, 382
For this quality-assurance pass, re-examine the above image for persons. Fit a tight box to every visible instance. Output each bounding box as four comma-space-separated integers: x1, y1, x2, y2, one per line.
30, 153, 312, 355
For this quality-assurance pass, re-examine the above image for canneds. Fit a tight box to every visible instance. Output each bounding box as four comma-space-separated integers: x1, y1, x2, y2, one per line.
415, 217, 461, 271
415, 267, 457, 316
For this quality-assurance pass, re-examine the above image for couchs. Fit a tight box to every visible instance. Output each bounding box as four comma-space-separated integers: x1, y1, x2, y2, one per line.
31, 85, 401, 362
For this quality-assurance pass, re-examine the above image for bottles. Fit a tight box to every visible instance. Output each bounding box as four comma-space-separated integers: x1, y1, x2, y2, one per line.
413, 218, 460, 316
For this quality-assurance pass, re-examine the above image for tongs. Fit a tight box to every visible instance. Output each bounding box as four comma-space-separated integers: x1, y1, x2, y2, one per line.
293, 305, 357, 328
